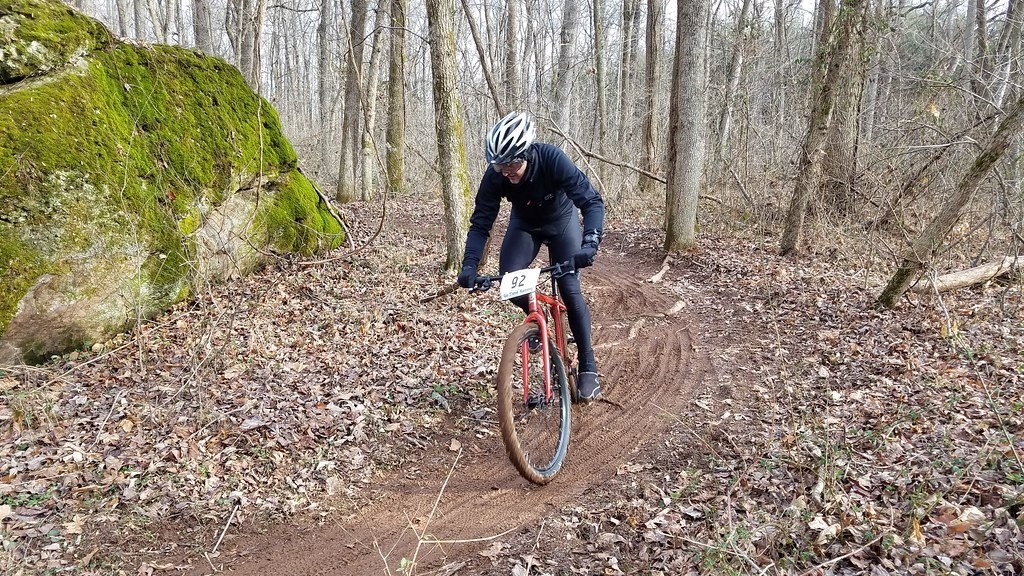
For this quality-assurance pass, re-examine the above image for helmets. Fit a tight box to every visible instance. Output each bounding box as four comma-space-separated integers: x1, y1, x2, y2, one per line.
482, 109, 536, 165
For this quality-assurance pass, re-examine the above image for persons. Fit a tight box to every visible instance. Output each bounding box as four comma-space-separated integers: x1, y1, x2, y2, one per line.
457, 111, 608, 401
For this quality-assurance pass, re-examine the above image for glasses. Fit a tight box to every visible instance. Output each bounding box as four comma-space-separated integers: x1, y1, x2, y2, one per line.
490, 162, 522, 175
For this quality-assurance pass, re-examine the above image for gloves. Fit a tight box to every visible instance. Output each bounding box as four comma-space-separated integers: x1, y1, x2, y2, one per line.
573, 242, 597, 269
456, 265, 476, 288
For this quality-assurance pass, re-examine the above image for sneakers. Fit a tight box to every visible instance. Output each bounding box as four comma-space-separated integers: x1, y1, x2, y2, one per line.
528, 310, 549, 354
576, 368, 601, 405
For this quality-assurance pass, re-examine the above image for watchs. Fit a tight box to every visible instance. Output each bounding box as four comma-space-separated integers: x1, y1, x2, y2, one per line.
581, 234, 600, 244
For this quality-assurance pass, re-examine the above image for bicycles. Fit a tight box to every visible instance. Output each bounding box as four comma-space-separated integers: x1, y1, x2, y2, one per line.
471, 240, 580, 486
